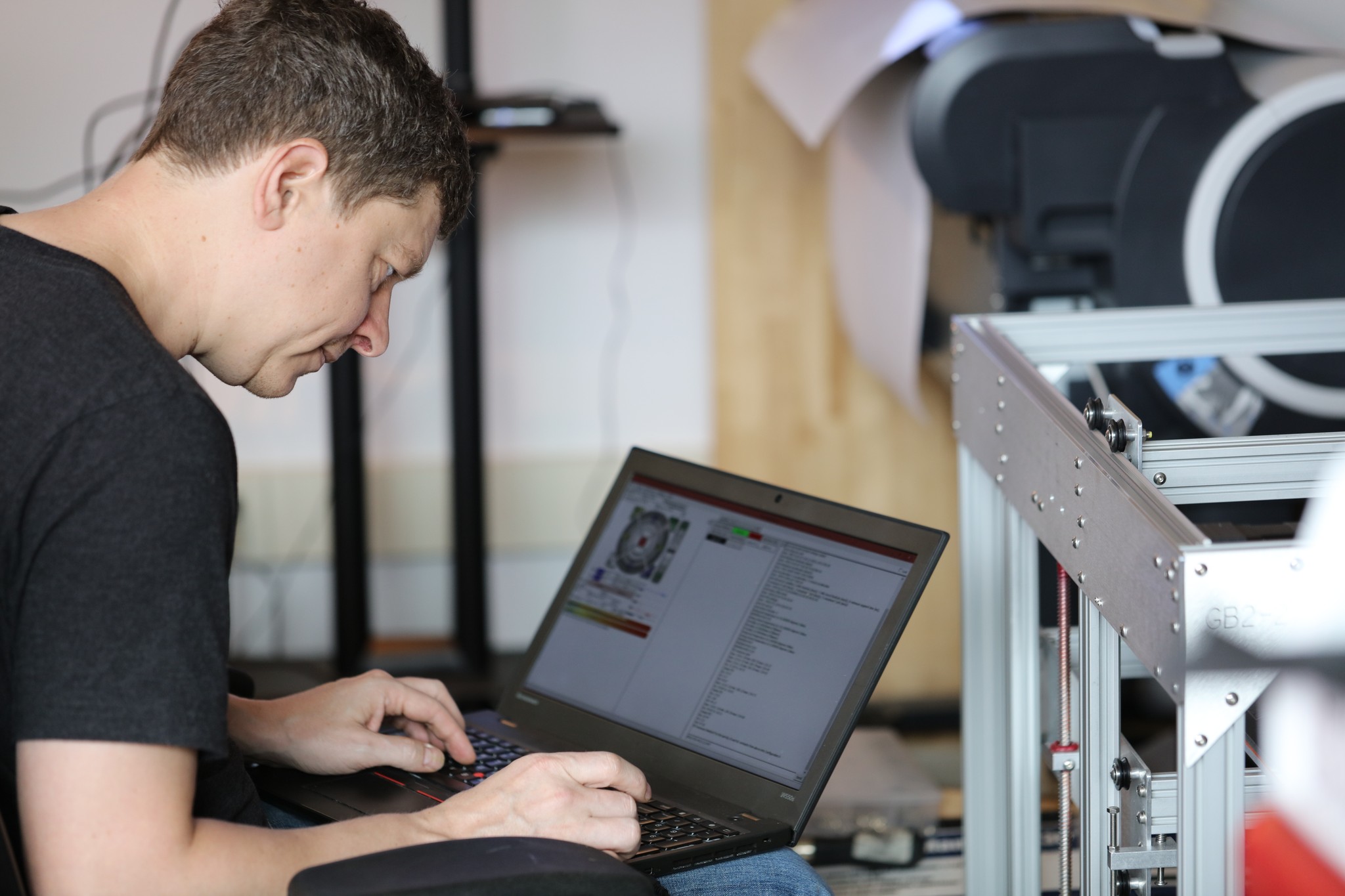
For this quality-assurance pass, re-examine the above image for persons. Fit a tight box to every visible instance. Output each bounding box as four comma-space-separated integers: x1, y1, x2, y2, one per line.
0, 0, 838, 896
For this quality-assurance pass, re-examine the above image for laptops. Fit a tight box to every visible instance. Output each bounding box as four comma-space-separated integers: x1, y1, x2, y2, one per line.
244, 448, 950, 876
